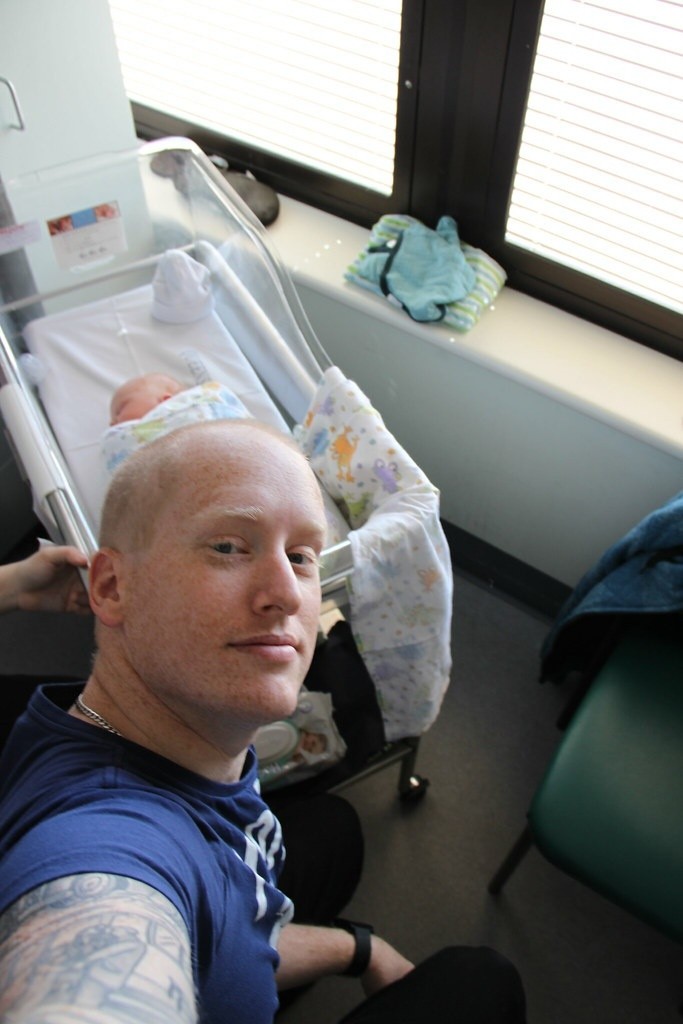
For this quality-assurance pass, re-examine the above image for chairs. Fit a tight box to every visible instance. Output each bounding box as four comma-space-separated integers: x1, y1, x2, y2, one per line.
487, 613, 682, 939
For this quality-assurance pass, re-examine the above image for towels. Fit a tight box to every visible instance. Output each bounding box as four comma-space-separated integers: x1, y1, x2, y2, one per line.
288, 363, 455, 747
343, 213, 509, 333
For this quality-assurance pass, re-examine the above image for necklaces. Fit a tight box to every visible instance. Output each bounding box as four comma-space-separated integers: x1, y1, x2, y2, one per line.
75, 695, 124, 738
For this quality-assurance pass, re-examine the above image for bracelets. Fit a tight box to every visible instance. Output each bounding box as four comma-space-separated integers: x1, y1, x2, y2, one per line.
331, 917, 375, 979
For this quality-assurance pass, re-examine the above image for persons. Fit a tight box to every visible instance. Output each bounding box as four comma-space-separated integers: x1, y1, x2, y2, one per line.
106, 373, 190, 446
0, 546, 94, 618
0, 416, 524, 1024
